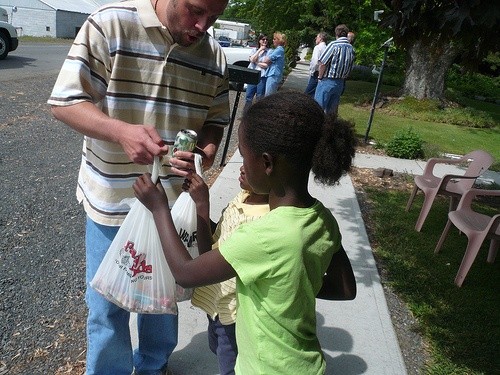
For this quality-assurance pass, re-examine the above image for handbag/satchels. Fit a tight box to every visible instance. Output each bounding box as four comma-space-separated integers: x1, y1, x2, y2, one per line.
90, 154, 178, 317
170, 153, 204, 302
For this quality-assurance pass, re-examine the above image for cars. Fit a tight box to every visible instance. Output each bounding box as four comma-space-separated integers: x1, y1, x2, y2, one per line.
0, 7, 19, 59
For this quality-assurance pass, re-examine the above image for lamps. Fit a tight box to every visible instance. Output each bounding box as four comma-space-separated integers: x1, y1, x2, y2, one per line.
12, 6, 17, 11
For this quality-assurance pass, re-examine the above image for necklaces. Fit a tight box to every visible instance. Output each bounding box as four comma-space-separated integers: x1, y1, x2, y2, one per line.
155, 0, 158, 10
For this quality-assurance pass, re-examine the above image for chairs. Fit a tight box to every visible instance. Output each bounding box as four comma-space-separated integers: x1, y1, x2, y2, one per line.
404, 149, 495, 235
435, 189, 500, 288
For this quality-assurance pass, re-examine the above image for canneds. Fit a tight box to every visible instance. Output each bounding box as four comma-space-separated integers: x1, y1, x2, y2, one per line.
170, 127, 197, 170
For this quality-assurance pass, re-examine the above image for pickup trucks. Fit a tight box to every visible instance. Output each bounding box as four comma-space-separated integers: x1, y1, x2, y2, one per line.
207, 26, 256, 89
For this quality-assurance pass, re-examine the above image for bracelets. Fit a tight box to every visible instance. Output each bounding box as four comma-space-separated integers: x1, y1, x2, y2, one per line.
318, 76, 323, 80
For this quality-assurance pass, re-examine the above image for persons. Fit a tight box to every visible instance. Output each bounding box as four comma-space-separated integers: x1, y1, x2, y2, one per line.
315, 24, 355, 118
263, 31, 287, 96
46, 0, 231, 375
305, 31, 329, 96
189, 163, 270, 375
238, 34, 271, 119
133, 92, 359, 375
341, 31, 355, 96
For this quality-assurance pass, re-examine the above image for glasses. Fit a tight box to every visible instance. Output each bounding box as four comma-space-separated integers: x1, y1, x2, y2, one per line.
261, 38, 268, 41
